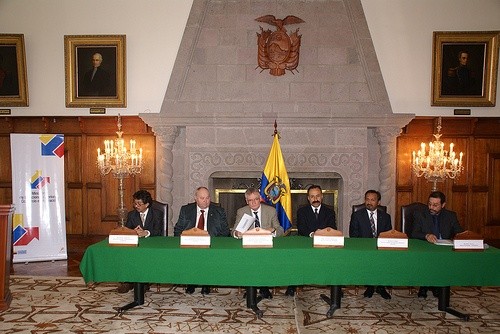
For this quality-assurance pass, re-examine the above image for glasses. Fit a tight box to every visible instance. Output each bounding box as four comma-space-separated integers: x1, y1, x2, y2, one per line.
133, 202, 146, 206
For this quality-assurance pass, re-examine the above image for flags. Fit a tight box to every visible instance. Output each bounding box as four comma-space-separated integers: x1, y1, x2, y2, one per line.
261, 135, 293, 235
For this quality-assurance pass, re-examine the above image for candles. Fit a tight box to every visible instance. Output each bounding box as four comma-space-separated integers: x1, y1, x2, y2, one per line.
413, 141, 463, 171
97, 137, 142, 168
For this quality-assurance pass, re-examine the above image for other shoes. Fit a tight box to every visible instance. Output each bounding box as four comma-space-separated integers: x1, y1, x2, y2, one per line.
375, 287, 391, 299
433, 292, 450, 300
363, 287, 375, 298
418, 289, 427, 298
117, 284, 134, 293
143, 284, 150, 293
185, 288, 194, 294
284, 288, 294, 297
200, 287, 210, 294
259, 290, 273, 299
242, 290, 257, 298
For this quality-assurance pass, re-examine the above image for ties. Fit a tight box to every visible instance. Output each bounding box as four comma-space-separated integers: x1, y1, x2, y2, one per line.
253, 212, 260, 228
315, 209, 318, 222
141, 214, 145, 222
434, 216, 439, 235
197, 210, 205, 230
369, 213, 376, 238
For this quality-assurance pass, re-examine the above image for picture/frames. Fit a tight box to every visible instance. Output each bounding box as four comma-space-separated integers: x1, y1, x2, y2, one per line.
63, 34, 128, 108
0, 33, 30, 108
430, 30, 500, 107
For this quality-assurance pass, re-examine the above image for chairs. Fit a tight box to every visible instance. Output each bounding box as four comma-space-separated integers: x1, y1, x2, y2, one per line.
402, 203, 428, 237
151, 200, 169, 236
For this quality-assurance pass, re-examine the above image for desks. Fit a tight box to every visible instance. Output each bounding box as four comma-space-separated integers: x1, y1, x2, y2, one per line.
80, 235, 500, 321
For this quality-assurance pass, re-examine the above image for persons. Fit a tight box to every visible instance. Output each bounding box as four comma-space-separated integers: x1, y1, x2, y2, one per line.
284, 185, 337, 296
349, 190, 392, 299
231, 187, 285, 301
411, 191, 464, 298
114, 191, 165, 294
174, 187, 230, 294
